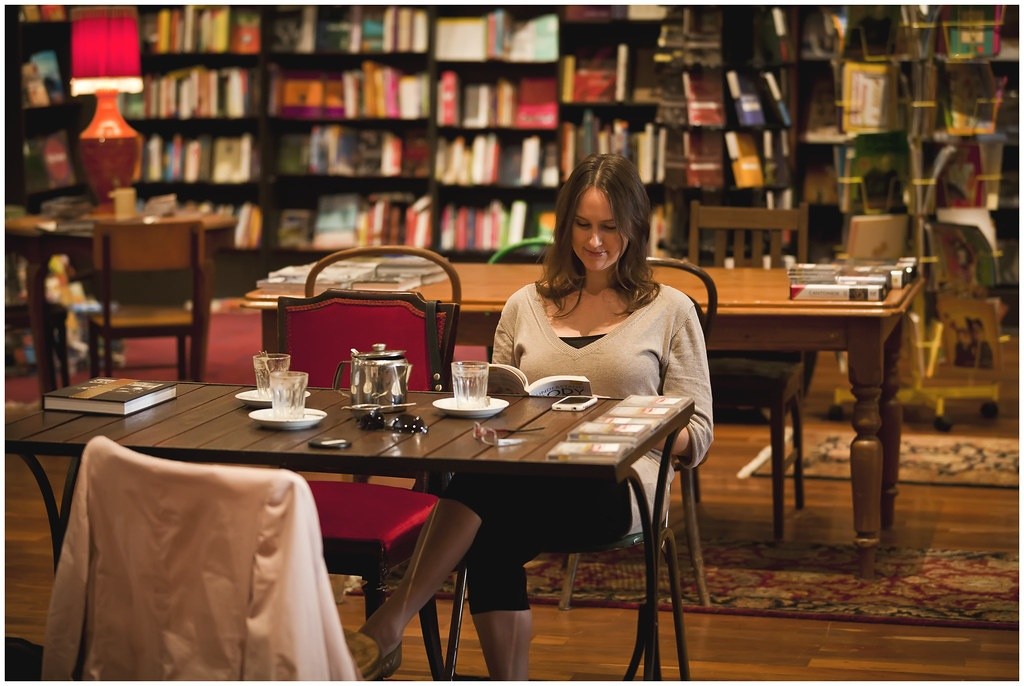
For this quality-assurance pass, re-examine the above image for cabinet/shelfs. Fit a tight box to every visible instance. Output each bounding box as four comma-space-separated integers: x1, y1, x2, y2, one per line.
5, 5, 1020, 256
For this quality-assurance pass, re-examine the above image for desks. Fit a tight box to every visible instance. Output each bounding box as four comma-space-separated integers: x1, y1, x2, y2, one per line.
240, 256, 926, 550
5, 383, 692, 682
5, 210, 236, 378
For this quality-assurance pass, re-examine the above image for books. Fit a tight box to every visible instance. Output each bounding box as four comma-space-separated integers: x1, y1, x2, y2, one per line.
4, 5, 1019, 373
489, 363, 594, 398
42, 377, 179, 418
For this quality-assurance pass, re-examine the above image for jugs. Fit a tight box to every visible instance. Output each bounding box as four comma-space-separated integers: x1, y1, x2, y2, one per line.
331, 343, 413, 415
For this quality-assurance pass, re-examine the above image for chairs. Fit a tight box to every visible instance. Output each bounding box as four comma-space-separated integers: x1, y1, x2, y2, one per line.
5, 206, 802, 682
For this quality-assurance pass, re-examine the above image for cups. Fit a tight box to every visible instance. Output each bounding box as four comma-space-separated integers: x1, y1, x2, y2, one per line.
270, 371, 309, 420
253, 354, 291, 400
451, 361, 490, 409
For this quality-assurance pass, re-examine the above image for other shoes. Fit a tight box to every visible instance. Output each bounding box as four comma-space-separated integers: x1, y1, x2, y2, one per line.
380, 641, 404, 678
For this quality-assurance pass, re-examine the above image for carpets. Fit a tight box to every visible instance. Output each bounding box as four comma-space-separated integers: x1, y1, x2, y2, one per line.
339, 533, 1019, 626
752, 429, 1019, 488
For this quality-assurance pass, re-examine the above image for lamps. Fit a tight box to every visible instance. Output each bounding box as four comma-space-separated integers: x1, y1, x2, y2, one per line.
70, 5, 143, 205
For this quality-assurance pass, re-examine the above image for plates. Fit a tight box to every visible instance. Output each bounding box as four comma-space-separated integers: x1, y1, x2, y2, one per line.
234, 390, 311, 408
248, 409, 327, 430
432, 397, 510, 418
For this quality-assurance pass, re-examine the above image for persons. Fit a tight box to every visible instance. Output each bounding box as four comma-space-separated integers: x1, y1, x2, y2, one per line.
343, 153, 716, 681
